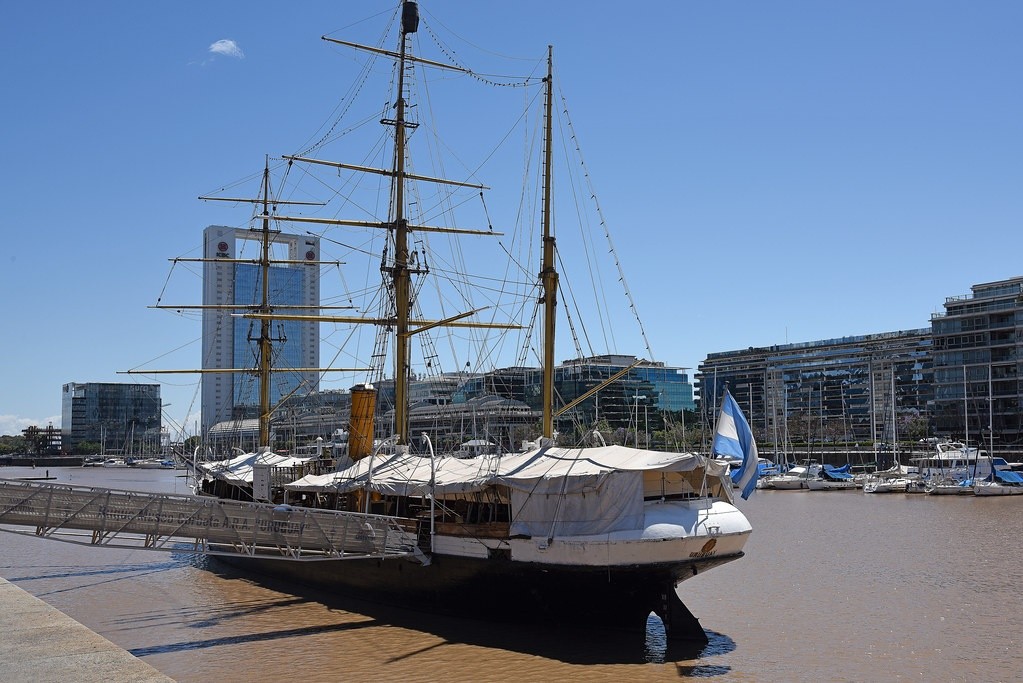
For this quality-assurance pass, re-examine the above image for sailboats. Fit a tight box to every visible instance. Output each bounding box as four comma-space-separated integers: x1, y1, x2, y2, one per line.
116, 0, 751, 615
1, 350, 1022, 495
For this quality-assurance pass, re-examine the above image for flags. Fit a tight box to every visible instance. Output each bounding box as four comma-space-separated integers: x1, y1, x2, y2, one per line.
712, 390, 759, 500
